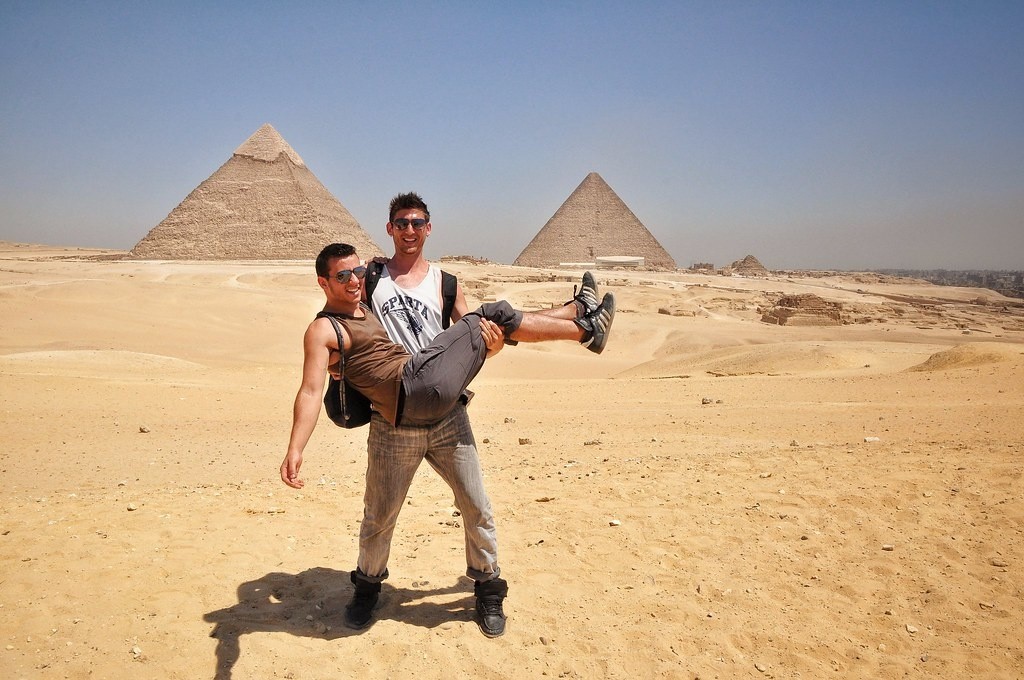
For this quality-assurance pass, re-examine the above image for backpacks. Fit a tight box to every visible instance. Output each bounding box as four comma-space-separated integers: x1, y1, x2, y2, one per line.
314, 303, 373, 429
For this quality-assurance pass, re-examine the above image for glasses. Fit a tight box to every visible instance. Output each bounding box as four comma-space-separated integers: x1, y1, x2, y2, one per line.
328, 266, 367, 284
392, 218, 426, 230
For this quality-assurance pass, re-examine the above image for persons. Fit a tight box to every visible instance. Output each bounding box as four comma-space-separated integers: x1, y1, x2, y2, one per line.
280, 243, 616, 489
328, 193, 506, 637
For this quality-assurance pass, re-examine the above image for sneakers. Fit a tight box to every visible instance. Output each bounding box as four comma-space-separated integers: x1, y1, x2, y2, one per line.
564, 270, 600, 318
343, 571, 381, 629
474, 578, 508, 638
574, 291, 615, 355
